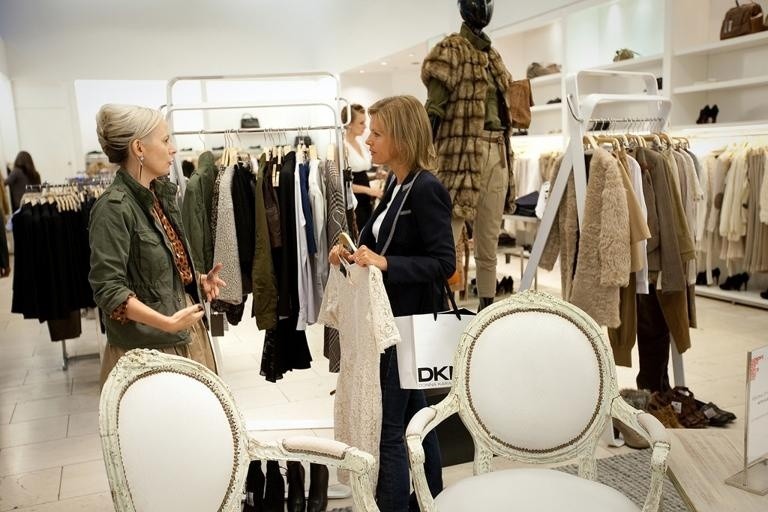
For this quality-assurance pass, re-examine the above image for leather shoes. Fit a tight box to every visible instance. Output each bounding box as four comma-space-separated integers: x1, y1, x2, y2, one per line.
243, 460, 329, 511
612, 384, 737, 449
3, 267, 9, 276
760, 288, 767, 299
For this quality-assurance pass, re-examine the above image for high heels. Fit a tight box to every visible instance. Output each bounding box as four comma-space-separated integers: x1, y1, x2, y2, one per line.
695, 268, 749, 291
696, 106, 710, 123
700, 104, 717, 123
471, 276, 514, 296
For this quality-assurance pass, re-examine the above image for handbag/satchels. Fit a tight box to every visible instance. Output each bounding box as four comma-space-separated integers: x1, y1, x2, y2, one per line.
613, 48, 638, 62
720, 0, 764, 41
240, 113, 259, 129
394, 257, 477, 391
526, 62, 561, 80
507, 77, 534, 129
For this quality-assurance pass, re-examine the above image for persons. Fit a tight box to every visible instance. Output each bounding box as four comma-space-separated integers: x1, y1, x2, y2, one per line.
86, 102, 229, 398
335, 103, 384, 229
421, 0, 512, 312
0, 150, 42, 210
327, 95, 458, 512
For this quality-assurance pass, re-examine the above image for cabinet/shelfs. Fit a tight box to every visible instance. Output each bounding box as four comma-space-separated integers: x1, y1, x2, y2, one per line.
665, 1, 768, 311
494, 10, 567, 136
560, 0, 665, 149
462, 209, 546, 297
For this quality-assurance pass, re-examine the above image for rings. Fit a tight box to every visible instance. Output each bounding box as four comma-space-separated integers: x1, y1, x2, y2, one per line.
354, 255, 361, 261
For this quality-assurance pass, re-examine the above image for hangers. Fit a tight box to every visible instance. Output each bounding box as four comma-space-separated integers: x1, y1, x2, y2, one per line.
713, 126, 768, 154
580, 117, 693, 151
21, 174, 116, 213
197, 125, 336, 169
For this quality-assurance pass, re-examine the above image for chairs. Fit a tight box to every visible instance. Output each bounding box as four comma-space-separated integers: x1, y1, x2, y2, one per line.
97, 348, 383, 512
403, 290, 673, 511
